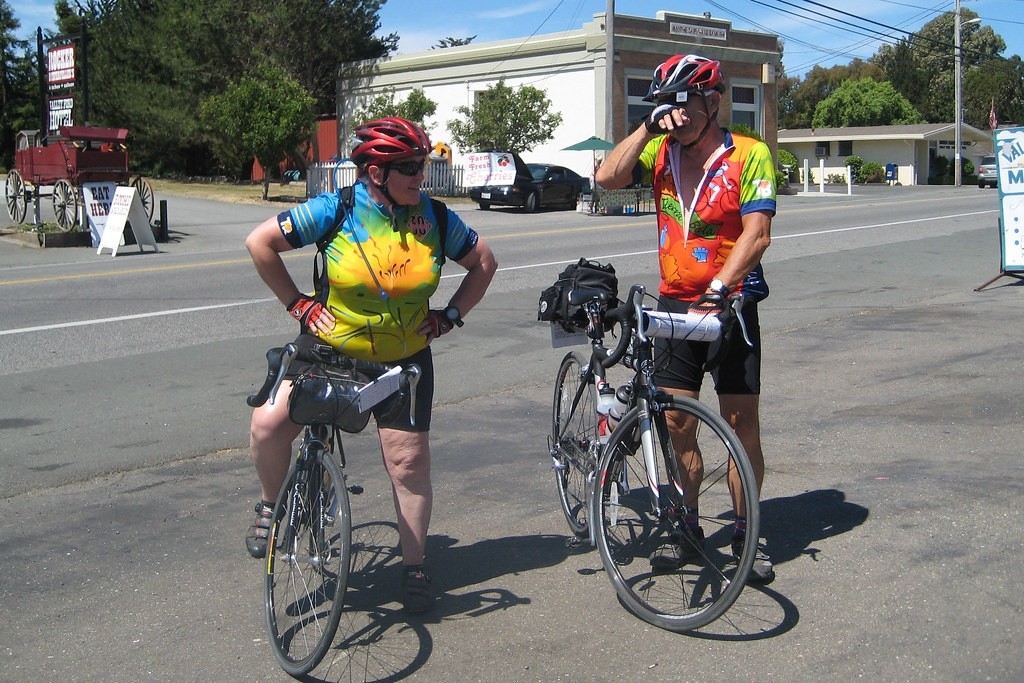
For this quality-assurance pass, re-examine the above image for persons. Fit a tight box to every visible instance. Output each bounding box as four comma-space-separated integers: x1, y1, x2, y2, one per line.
246, 120, 497, 612
596, 54, 778, 581
589, 154, 603, 213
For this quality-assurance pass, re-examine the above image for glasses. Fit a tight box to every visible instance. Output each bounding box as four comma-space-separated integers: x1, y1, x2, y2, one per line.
373, 159, 428, 176
657, 91, 699, 108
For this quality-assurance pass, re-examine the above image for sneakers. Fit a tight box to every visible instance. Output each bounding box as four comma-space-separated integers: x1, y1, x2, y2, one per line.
401, 573, 437, 614
246, 502, 284, 559
648, 525, 705, 568
731, 538, 773, 582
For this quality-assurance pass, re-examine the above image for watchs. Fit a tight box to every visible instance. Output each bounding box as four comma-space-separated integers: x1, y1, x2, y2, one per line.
444, 305, 464, 328
708, 279, 730, 299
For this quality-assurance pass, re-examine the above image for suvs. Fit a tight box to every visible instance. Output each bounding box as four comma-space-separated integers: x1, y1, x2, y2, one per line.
978, 155, 998, 188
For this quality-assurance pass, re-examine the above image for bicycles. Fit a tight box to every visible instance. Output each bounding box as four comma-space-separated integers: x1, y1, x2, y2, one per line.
244, 341, 423, 679
545, 284, 763, 633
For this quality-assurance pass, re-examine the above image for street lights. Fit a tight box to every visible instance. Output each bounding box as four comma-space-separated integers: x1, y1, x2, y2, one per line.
954, 17, 983, 188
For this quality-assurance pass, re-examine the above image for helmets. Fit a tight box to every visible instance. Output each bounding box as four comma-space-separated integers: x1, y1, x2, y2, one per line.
349, 116, 433, 168
642, 54, 727, 104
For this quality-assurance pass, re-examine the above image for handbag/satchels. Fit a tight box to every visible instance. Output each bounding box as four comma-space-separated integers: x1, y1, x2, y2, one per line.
539, 259, 617, 335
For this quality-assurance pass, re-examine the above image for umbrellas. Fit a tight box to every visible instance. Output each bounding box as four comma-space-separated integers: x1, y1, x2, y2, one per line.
561, 135, 617, 181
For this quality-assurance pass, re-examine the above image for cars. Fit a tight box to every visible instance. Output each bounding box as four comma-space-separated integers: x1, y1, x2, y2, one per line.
466, 149, 584, 213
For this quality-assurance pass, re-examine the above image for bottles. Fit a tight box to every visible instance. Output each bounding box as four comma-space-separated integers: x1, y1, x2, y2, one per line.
597, 383, 615, 444
607, 385, 631, 433
305, 379, 362, 400
